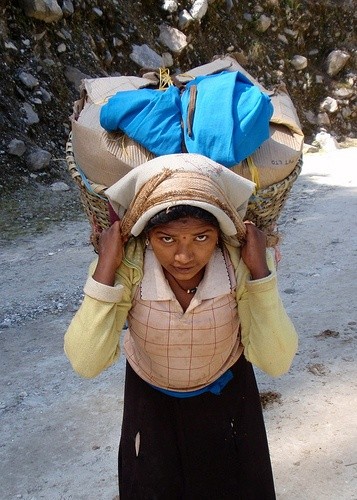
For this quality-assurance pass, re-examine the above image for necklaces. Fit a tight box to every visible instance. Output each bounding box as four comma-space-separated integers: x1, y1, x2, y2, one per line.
170, 266, 206, 294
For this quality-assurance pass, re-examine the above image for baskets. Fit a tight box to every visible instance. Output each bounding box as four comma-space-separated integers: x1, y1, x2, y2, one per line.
66, 131, 303, 256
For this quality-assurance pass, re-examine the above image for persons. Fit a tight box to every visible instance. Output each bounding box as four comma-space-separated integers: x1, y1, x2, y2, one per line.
64, 153, 299, 500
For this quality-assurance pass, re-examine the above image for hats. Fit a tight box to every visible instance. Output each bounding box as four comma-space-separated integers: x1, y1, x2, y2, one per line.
104, 153, 256, 236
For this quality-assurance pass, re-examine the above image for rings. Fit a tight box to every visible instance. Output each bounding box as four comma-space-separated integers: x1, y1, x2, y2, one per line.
243, 220, 256, 226
